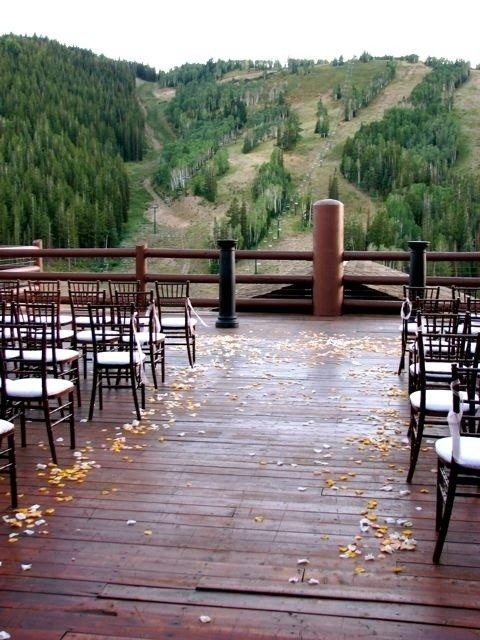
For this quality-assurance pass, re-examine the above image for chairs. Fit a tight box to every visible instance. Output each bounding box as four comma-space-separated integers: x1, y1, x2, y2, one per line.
67, 277, 196, 422
1, 276, 82, 509
396, 282, 480, 567
